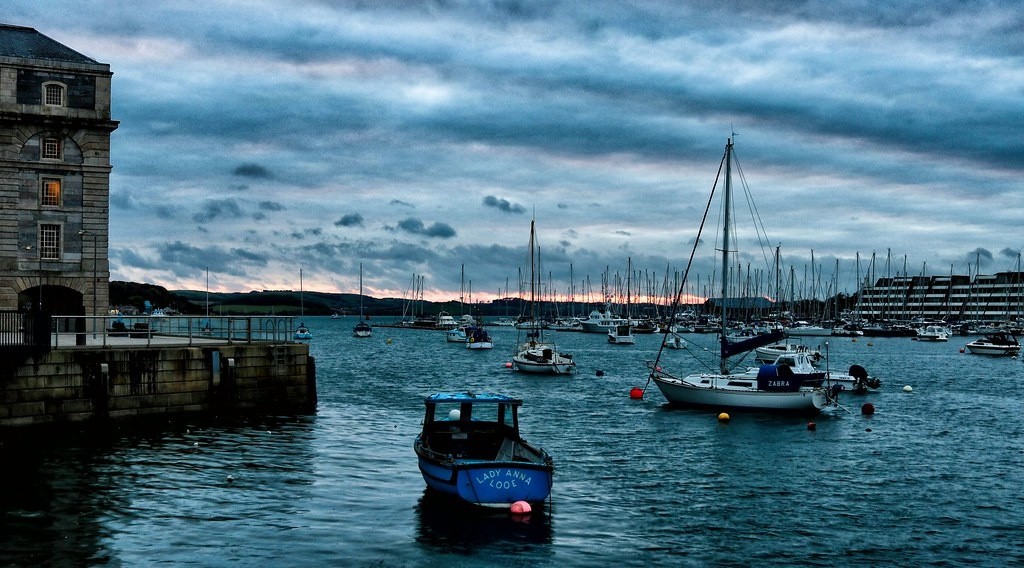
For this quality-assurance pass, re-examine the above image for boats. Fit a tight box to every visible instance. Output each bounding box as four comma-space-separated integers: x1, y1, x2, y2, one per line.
813, 370, 866, 392
414, 387, 556, 509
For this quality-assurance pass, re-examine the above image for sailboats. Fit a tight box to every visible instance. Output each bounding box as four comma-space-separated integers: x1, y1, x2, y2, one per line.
354, 261, 373, 338
512, 219, 575, 376
201, 266, 214, 335
754, 246, 824, 364
646, 120, 832, 413
375, 242, 1024, 356
294, 268, 313, 340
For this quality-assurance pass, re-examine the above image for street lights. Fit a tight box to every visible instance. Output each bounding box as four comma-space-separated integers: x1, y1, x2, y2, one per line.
79, 229, 97, 339
26, 246, 43, 310
